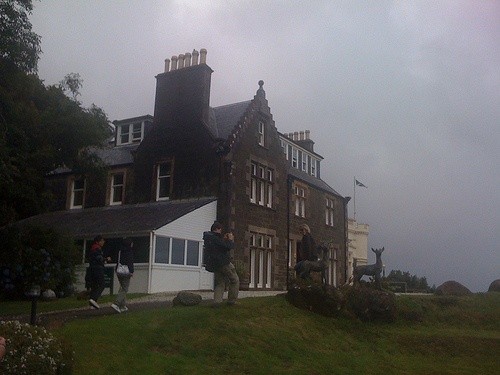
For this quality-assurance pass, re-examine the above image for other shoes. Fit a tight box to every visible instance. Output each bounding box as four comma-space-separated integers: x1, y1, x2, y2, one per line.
89, 298, 100, 310
227, 302, 241, 308
111, 303, 121, 313
213, 302, 225, 307
121, 306, 129, 312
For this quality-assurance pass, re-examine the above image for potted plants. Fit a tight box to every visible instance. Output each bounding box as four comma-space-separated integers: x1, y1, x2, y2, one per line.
236, 265, 250, 290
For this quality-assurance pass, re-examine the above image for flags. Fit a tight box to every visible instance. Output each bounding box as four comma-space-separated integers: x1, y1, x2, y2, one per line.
356, 179, 367, 188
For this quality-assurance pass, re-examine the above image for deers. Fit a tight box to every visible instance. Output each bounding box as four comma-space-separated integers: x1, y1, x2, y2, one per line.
295, 244, 338, 287
354, 247, 387, 291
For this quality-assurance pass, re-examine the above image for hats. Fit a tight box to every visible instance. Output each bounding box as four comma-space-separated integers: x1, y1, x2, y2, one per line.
211, 222, 224, 229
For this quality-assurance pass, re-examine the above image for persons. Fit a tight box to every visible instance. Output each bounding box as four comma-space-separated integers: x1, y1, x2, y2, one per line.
89, 235, 111, 309
295, 224, 320, 279
203, 222, 240, 305
111, 239, 134, 312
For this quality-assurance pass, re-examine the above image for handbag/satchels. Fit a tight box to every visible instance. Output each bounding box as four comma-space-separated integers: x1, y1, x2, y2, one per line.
117, 264, 130, 277
